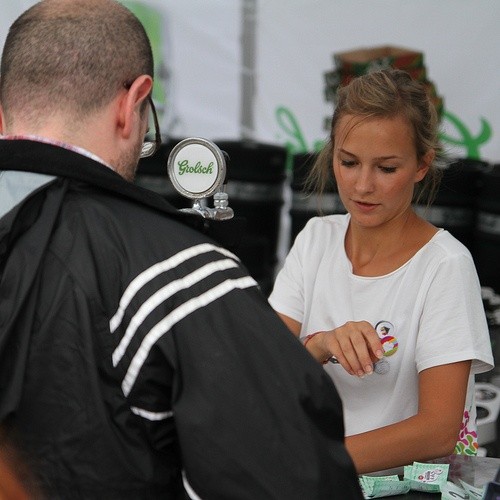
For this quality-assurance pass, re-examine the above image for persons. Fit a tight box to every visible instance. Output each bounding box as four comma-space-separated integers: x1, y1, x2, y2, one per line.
0, 2, 365, 500
266, 45, 493, 474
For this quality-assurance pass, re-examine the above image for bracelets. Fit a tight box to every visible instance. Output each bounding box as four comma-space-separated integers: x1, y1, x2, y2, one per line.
300, 331, 332, 366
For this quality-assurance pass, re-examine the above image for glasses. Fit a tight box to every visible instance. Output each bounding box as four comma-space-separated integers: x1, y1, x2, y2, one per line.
123, 81, 161, 159
